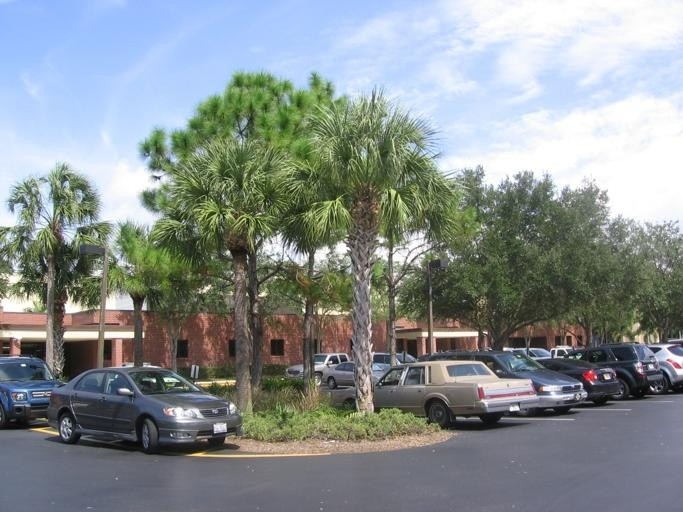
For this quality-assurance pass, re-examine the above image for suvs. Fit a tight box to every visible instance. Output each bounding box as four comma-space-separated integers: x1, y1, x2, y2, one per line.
0, 351, 66, 430
284, 352, 350, 387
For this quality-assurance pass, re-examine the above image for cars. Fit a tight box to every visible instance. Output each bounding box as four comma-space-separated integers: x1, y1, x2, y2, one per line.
45, 365, 246, 456
325, 357, 542, 430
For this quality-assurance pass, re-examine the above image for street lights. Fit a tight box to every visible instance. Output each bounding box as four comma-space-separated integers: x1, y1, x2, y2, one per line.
77, 242, 109, 369
426, 257, 451, 356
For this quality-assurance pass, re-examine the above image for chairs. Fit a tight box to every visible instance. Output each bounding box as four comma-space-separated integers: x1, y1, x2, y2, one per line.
84, 379, 98, 392
